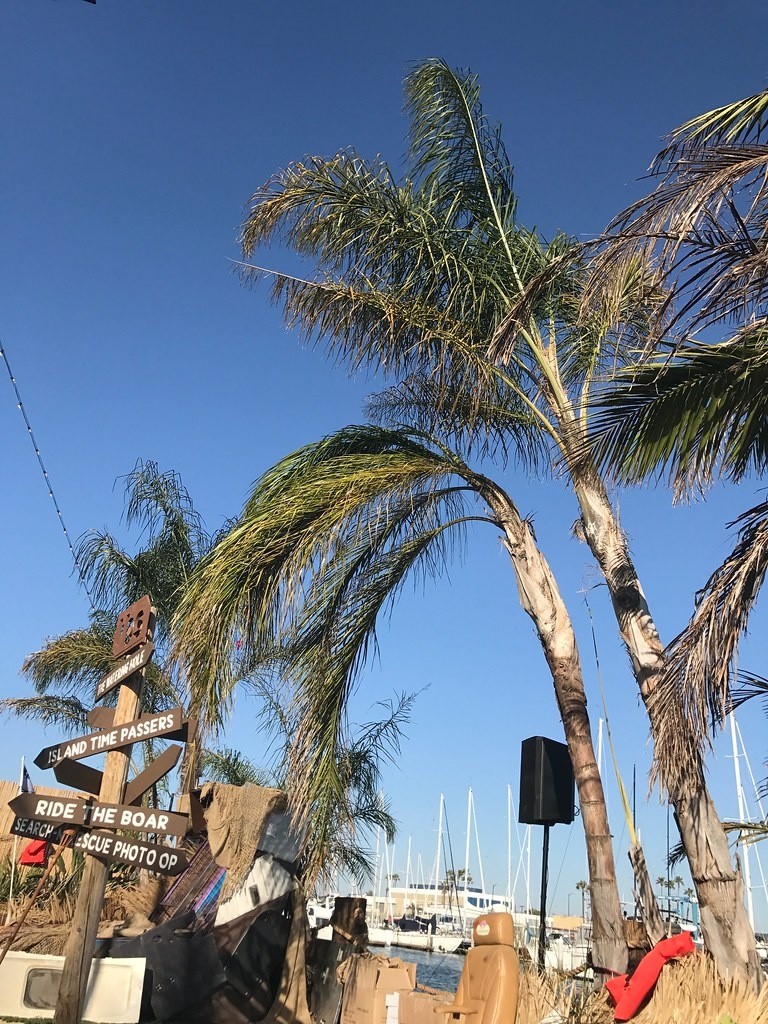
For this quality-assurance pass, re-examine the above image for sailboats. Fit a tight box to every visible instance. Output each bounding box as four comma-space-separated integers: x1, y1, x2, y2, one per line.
305, 693, 767, 982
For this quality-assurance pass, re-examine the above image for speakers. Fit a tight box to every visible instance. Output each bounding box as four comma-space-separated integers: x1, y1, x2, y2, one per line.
518, 736, 576, 827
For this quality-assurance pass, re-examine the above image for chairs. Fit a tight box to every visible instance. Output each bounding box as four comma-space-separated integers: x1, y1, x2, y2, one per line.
432, 912, 520, 1024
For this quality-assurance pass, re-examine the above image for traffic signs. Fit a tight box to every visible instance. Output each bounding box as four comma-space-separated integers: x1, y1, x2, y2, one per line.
8, 791, 190, 838
9, 814, 190, 878
32, 707, 183, 770
94, 642, 156, 703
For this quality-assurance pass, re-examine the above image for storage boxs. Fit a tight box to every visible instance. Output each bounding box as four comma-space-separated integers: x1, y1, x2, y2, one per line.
339, 958, 455, 1024
